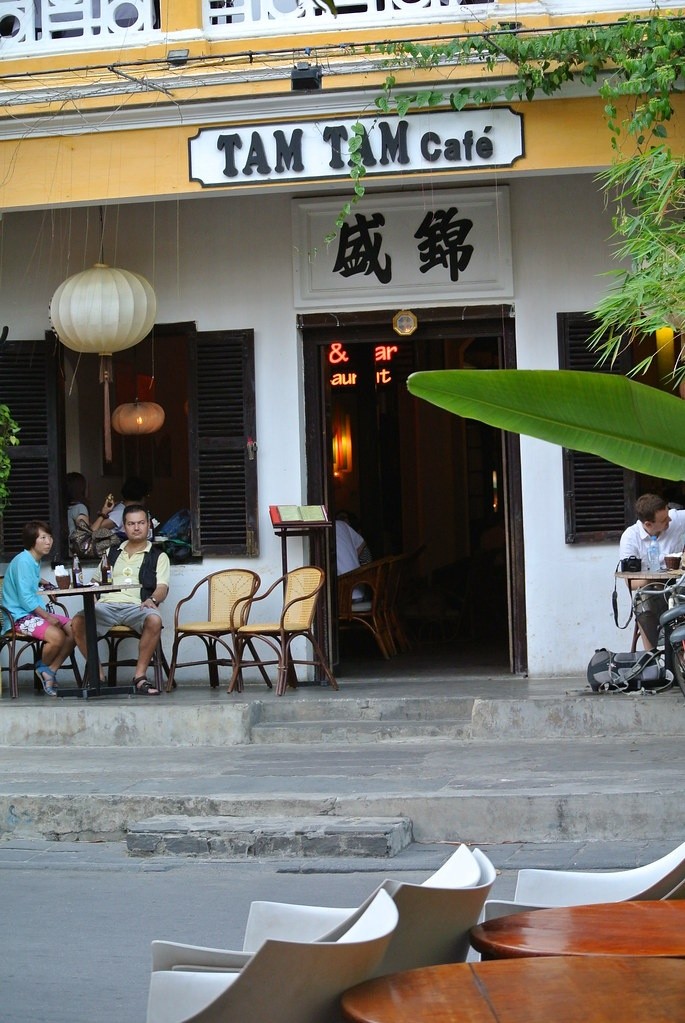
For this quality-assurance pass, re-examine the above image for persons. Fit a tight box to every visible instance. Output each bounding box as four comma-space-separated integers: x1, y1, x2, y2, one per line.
71, 504, 170, 695
3, 520, 77, 696
65, 472, 115, 559
334, 508, 373, 658
100, 476, 153, 541
620, 495, 685, 651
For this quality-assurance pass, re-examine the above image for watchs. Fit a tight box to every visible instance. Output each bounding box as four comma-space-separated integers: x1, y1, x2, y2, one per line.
149, 597, 157, 606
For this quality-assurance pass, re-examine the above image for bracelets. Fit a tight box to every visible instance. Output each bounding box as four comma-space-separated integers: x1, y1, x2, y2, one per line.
98, 513, 108, 519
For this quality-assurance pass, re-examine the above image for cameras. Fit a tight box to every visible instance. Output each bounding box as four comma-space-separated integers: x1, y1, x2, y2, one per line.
621, 556, 642, 572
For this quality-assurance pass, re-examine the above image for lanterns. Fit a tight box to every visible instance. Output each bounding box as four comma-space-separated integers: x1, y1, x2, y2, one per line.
111, 401, 165, 435
49, 263, 158, 356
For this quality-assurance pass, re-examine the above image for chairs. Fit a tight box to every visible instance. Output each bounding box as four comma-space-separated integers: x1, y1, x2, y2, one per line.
147, 844, 496, 1023
479, 843, 685, 963
166, 566, 339, 695
0, 576, 83, 698
95, 593, 177, 691
337, 553, 411, 661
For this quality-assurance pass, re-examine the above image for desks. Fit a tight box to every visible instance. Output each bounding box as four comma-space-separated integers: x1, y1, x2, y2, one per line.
615, 571, 685, 686
341, 955, 685, 1023
470, 899, 685, 962
273, 522, 333, 687
37, 584, 142, 699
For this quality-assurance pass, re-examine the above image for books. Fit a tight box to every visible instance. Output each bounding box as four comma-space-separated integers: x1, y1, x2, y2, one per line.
276, 505, 326, 522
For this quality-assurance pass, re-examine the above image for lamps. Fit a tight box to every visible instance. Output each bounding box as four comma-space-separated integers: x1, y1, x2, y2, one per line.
498, 22, 522, 36
291, 48, 324, 92
167, 49, 189, 66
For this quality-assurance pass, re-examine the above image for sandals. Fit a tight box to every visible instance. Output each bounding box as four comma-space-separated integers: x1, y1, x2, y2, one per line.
131, 676, 160, 696
34, 660, 59, 696
100, 676, 110, 688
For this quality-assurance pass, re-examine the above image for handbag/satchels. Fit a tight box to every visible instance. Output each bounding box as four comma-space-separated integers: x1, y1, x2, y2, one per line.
68, 521, 114, 559
160, 509, 192, 543
587, 647, 675, 696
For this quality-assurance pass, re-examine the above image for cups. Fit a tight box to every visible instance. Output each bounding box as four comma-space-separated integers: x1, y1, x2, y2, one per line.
665, 556, 681, 570
56, 573, 71, 589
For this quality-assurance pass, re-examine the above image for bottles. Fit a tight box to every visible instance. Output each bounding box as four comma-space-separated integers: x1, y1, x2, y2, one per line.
73, 553, 84, 588
647, 536, 660, 571
100, 551, 112, 585
